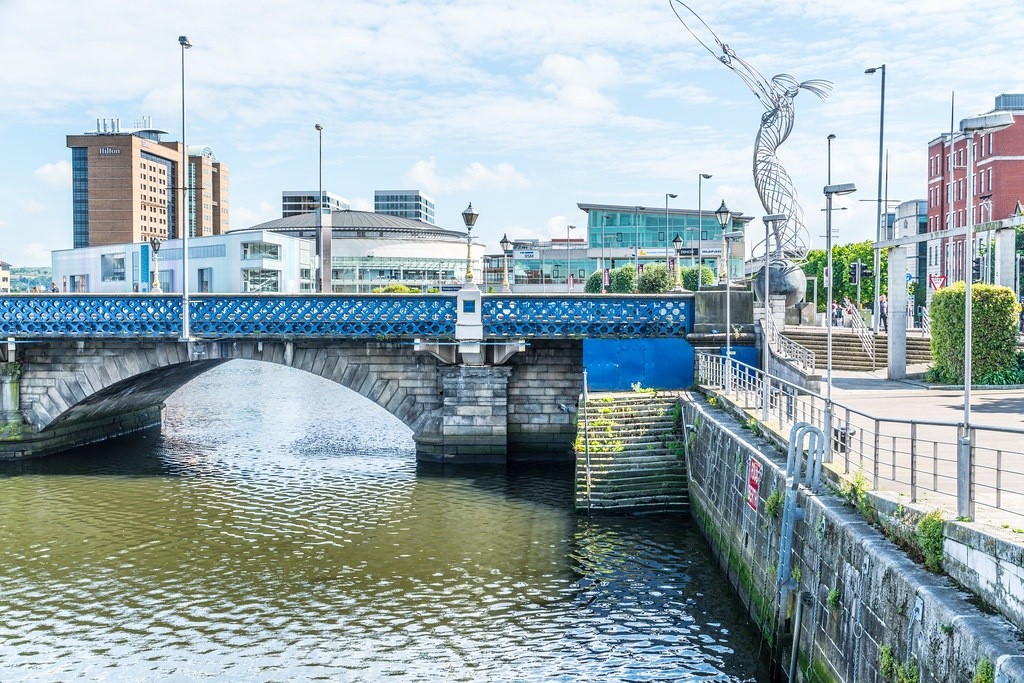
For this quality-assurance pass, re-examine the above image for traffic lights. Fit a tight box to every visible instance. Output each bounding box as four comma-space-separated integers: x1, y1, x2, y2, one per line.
918, 306, 922, 316
972, 257, 983, 282
848, 263, 856, 285
861, 263, 872, 277
1020, 256, 1024, 271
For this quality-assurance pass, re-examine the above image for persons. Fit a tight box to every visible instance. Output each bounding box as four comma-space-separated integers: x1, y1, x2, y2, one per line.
51, 283, 59, 292
880, 294, 888, 333
833, 295, 853, 326
151, 282, 162, 293
34, 286, 40, 292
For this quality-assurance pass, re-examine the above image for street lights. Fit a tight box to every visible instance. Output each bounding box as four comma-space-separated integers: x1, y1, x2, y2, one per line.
672, 233, 684, 292
823, 208, 848, 325
686, 226, 698, 266
151, 236, 162, 293
178, 36, 193, 336
602, 214, 612, 289
635, 205, 646, 269
823, 183, 857, 460
958, 113, 1016, 517
864, 66, 886, 332
567, 225, 576, 291
715, 199, 732, 285
724, 230, 744, 395
499, 233, 512, 293
462, 202, 479, 285
315, 123, 323, 293
665, 193, 678, 270
763, 213, 787, 419
698, 172, 713, 290
828, 133, 835, 184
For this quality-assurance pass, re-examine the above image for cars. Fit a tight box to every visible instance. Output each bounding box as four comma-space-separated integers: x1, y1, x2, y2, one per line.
915, 321, 922, 327
